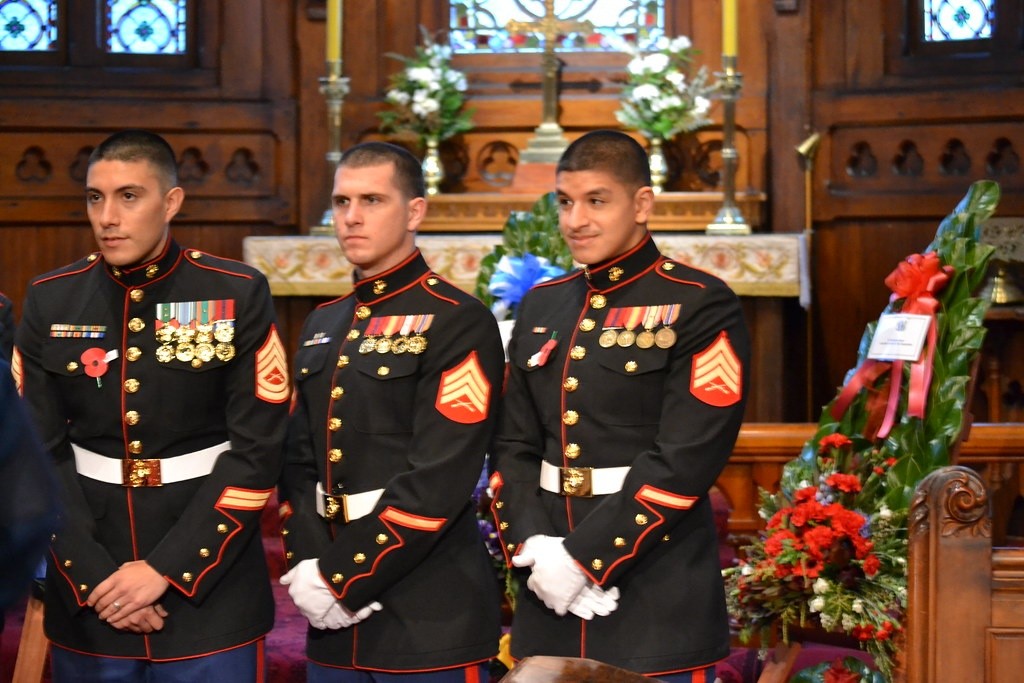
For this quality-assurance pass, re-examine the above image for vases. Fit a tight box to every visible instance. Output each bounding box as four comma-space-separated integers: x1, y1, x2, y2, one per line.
417, 137, 445, 194
647, 138, 673, 197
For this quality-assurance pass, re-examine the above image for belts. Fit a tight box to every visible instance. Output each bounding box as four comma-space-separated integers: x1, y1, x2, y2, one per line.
537, 459, 632, 497
315, 482, 385, 522
72, 440, 232, 488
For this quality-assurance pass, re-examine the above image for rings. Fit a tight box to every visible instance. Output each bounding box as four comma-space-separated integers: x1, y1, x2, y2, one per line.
113, 602, 121, 610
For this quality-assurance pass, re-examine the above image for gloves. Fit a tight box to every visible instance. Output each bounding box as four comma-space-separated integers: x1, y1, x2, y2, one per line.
512, 533, 620, 619
510, 536, 588, 615
280, 556, 339, 619
309, 602, 384, 630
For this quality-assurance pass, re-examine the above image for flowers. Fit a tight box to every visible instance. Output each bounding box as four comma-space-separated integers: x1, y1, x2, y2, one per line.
376, 24, 479, 144
714, 179, 1004, 683
616, 25, 716, 137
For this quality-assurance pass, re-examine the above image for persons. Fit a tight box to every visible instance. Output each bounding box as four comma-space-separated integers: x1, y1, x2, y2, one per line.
276, 141, 506, 683
486, 130, 752, 683
0, 134, 291, 683
